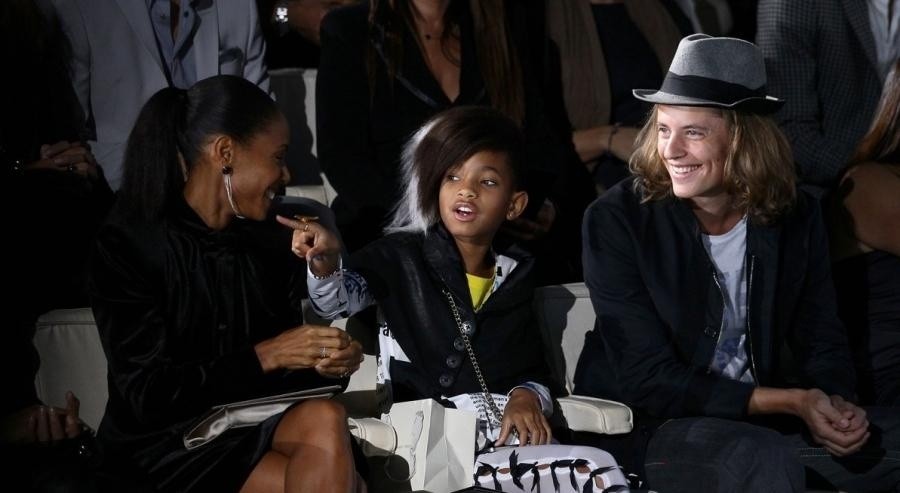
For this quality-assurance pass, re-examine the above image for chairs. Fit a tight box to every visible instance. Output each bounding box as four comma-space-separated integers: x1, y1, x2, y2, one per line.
269, 70, 337, 207
31, 282, 634, 460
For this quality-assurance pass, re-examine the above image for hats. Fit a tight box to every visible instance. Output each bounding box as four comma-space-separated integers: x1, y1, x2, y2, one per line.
630, 28, 789, 116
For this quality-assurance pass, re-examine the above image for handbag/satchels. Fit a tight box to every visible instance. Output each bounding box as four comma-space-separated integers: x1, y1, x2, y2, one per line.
442, 391, 558, 452
182, 382, 343, 452
